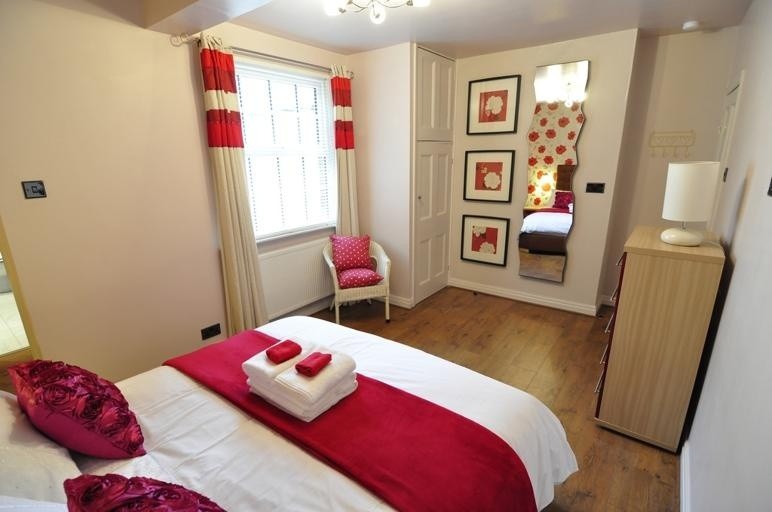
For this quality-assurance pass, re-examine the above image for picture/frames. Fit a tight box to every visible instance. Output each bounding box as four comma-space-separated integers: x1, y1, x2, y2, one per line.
463, 150, 515, 203
466, 74, 521, 135
461, 214, 510, 266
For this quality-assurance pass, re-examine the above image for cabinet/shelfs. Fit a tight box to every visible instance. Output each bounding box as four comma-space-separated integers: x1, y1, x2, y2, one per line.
593, 225, 725, 452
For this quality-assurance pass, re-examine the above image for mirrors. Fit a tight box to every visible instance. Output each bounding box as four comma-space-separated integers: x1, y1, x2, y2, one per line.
518, 59, 589, 282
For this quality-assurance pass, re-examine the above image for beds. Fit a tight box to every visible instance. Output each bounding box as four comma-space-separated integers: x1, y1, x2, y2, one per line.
517, 164, 576, 255
0, 316, 578, 512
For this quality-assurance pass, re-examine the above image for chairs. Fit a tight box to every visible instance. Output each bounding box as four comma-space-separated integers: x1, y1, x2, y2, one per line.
323, 241, 391, 325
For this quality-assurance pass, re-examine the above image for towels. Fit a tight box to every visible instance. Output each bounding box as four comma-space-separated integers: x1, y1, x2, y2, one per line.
241, 336, 358, 423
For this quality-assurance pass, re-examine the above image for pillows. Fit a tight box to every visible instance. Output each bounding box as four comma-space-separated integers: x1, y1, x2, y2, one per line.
0, 390, 82, 512
337, 268, 384, 289
547, 188, 574, 209
329, 232, 374, 271
64, 474, 227, 512
7, 358, 147, 459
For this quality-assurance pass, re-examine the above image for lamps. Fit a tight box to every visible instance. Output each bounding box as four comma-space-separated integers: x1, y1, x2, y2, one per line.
322, 0, 430, 24
659, 160, 720, 246
